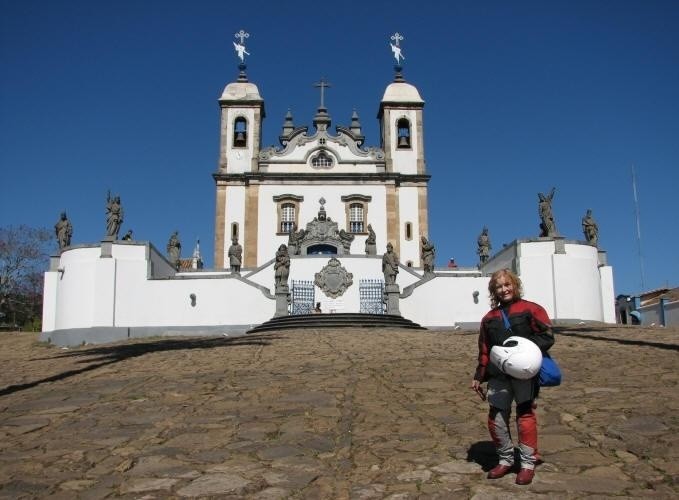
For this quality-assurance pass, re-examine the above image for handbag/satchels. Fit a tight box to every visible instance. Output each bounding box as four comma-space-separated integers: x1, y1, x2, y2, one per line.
537, 349, 562, 387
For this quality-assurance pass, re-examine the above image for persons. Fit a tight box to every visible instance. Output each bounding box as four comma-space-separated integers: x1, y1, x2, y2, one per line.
54, 211, 73, 249
470, 268, 556, 485
477, 224, 492, 265
104, 190, 124, 238
537, 186, 557, 236
166, 231, 181, 265
228, 236, 243, 273
381, 241, 399, 284
315, 302, 321, 313
420, 235, 435, 272
122, 230, 132, 241
274, 243, 290, 287
448, 257, 457, 267
365, 224, 377, 252
581, 208, 598, 246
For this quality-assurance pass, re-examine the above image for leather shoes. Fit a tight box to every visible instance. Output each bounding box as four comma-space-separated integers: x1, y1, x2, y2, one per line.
515, 468, 534, 484
488, 464, 512, 479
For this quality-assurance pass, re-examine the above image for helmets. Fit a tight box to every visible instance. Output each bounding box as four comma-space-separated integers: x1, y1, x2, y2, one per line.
490, 335, 543, 383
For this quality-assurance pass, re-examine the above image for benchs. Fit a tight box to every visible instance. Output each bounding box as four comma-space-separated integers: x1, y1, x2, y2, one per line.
0, 325, 22, 331
